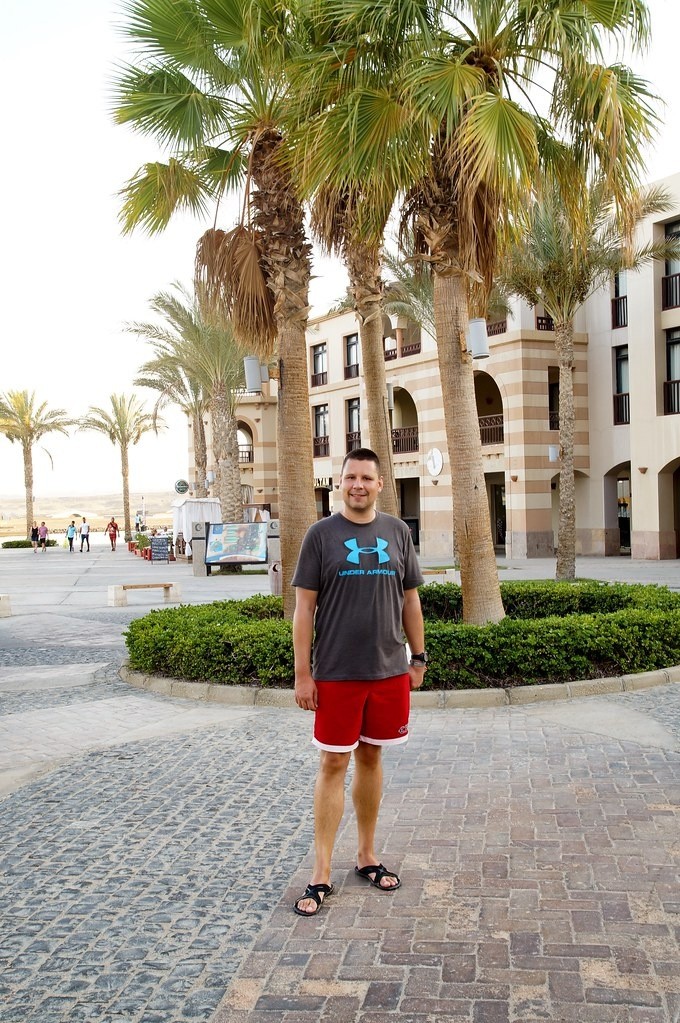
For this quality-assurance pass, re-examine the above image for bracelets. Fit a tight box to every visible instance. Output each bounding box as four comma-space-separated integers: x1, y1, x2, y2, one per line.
410, 660, 426, 667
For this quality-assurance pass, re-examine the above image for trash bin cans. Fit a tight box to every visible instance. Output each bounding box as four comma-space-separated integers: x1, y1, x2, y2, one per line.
267, 519, 283, 596
191, 521, 212, 577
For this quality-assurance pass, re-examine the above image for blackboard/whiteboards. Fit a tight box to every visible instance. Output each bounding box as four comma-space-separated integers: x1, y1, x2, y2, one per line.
151, 537, 169, 560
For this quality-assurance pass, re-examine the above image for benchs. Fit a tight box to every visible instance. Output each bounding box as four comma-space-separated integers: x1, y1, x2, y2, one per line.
109, 582, 180, 607
128, 541, 175, 560
0, 594, 12, 617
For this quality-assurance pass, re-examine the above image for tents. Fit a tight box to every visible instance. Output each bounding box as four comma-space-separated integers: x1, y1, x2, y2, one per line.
171, 498, 223, 557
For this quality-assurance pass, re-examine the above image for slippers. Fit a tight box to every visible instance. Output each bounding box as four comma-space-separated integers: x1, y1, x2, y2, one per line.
355, 863, 402, 890
293, 883, 334, 916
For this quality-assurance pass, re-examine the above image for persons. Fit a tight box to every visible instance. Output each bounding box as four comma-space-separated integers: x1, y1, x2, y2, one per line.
104, 517, 120, 551
29, 521, 39, 553
134, 513, 140, 532
290, 448, 428, 916
37, 522, 49, 552
65, 521, 77, 553
80, 517, 90, 552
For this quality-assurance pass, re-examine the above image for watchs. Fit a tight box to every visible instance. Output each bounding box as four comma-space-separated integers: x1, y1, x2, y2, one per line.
411, 651, 428, 662
